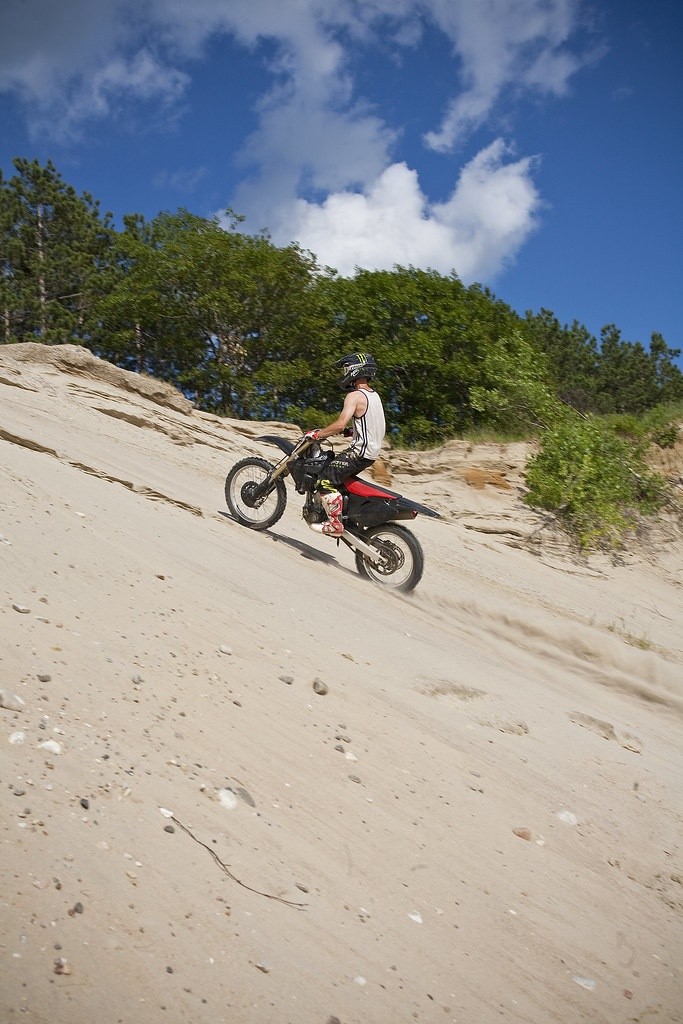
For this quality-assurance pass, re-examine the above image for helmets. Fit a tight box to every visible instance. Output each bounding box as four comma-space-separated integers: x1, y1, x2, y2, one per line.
336, 352, 377, 390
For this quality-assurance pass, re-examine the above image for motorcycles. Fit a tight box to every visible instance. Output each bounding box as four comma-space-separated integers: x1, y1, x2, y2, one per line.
223, 430, 443, 596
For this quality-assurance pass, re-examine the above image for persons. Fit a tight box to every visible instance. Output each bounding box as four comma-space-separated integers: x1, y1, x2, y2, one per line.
303, 352, 386, 536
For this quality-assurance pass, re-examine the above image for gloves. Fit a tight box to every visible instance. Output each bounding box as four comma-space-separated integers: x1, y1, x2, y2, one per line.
304, 429, 324, 440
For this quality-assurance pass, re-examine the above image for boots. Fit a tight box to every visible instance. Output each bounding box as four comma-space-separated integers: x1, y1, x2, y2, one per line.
310, 492, 344, 537
